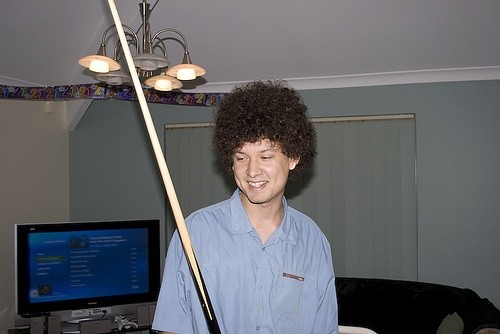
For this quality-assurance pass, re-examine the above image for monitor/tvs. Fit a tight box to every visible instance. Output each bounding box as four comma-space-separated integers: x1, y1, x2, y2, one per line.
16, 217, 166, 315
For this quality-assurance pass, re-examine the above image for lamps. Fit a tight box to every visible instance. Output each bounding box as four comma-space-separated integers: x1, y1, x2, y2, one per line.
78, 0, 207, 92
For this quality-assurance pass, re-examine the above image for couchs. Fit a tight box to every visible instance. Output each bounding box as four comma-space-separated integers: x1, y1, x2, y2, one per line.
334, 277, 500, 334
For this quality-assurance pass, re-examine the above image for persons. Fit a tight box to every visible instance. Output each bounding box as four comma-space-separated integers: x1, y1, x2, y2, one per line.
151, 81, 338, 334
